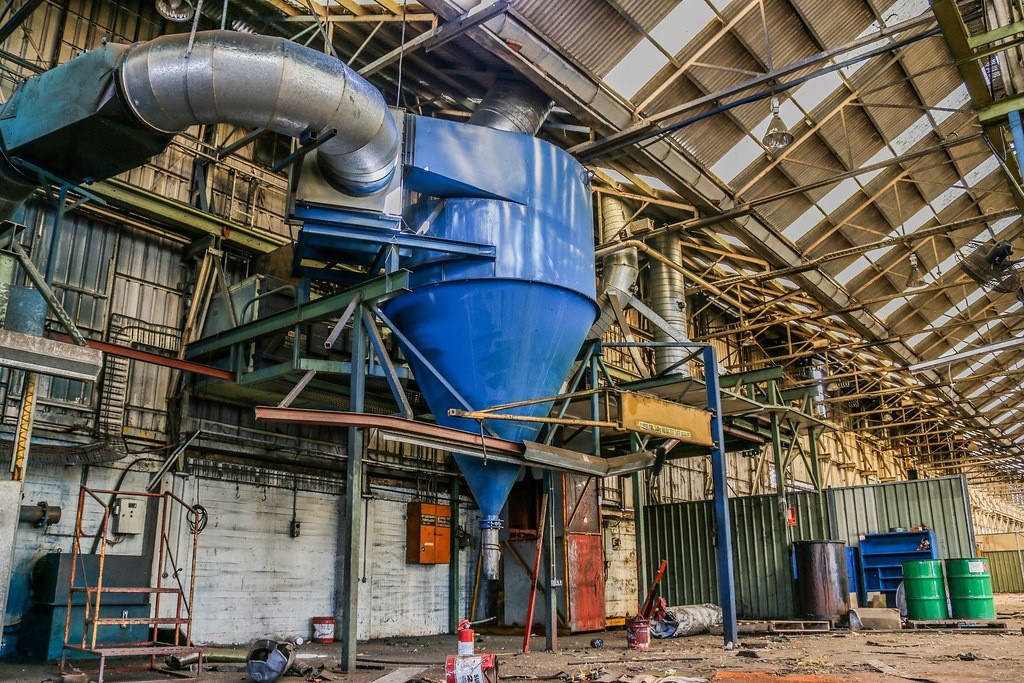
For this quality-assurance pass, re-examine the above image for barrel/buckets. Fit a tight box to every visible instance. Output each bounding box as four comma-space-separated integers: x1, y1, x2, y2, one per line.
793, 540, 851, 621
4, 284, 48, 337
900, 559, 948, 619
944, 558, 997, 620
313, 616, 334, 644
445, 654, 498, 683
625, 617, 651, 650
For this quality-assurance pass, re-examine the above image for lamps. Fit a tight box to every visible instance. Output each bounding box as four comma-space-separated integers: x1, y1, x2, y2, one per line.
906, 253, 927, 288
742, 319, 757, 346
760, 0, 796, 149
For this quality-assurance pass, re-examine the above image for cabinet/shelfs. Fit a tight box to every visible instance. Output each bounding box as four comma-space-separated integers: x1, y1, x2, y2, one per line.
859, 529, 939, 608
405, 502, 452, 566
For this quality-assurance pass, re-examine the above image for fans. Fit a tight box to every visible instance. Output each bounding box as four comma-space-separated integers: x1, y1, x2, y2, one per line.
954, 238, 1024, 303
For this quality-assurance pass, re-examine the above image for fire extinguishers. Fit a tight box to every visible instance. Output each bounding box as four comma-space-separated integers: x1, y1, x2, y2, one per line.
457, 620, 474, 656
